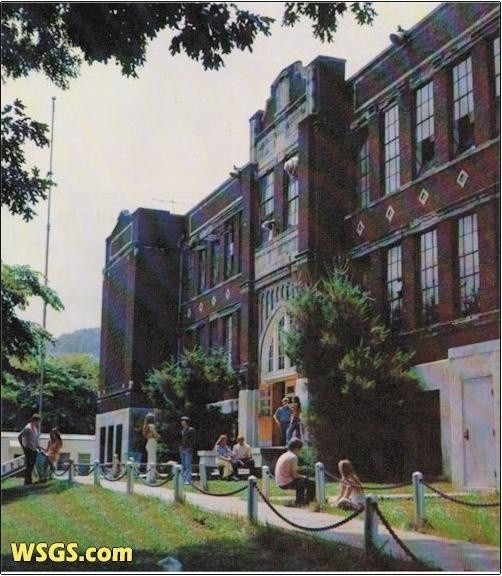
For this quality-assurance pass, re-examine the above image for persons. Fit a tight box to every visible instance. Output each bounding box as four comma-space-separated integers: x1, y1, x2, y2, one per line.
178, 414, 196, 485
141, 412, 160, 484
38, 427, 64, 482
213, 434, 246, 481
284, 395, 303, 445
232, 434, 258, 479
272, 396, 292, 446
274, 435, 316, 505
326, 457, 366, 512
17, 412, 41, 485
